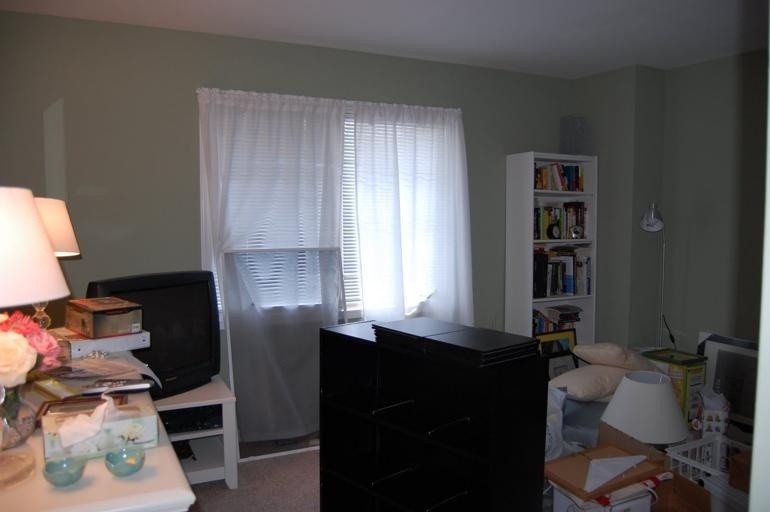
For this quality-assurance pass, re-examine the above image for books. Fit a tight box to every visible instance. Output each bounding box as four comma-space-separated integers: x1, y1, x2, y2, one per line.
534, 161, 590, 335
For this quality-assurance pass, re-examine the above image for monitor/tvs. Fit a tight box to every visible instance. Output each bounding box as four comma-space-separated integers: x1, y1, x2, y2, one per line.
85, 270, 221, 402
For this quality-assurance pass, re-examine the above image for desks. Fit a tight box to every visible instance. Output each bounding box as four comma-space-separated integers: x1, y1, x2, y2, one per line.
0, 327, 197, 512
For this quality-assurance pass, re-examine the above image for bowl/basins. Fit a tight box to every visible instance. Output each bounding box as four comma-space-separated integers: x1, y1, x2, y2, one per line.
43, 455, 88, 489
104, 446, 146, 478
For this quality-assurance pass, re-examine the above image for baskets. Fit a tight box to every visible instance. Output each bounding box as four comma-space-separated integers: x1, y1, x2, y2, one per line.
665, 436, 752, 488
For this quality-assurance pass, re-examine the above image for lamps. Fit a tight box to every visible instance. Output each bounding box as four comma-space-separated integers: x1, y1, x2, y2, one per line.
639, 202, 665, 348
32, 197, 81, 329
601, 371, 689, 444
0, 185, 71, 310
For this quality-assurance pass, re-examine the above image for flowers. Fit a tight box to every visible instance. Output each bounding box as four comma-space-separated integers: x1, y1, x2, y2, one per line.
0, 310, 62, 426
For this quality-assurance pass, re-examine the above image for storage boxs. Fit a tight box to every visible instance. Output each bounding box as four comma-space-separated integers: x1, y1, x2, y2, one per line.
640, 348, 708, 426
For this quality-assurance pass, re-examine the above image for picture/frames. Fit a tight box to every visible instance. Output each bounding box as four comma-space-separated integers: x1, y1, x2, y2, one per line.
544, 351, 579, 380
544, 444, 666, 502
535, 328, 579, 366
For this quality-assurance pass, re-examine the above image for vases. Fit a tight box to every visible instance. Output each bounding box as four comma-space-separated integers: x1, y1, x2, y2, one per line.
0, 403, 38, 450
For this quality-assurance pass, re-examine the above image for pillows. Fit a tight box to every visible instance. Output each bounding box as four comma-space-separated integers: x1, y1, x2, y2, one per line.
548, 365, 628, 403
574, 343, 650, 369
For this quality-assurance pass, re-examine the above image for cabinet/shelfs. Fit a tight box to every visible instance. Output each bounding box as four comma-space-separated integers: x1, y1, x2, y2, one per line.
505, 151, 597, 366
154, 375, 238, 490
319, 323, 547, 512
